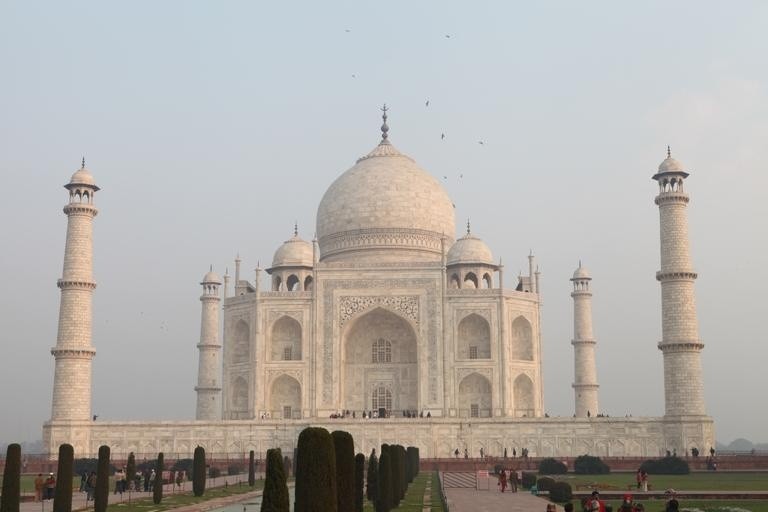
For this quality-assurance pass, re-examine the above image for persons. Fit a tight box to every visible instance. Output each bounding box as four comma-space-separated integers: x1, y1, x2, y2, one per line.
79, 469, 89, 492
636, 469, 642, 492
666, 448, 670, 456
498, 469, 507, 491
510, 469, 518, 493
176, 472, 182, 487
330, 409, 372, 418
546, 491, 679, 512
710, 447, 715, 456
45, 473, 55, 503
34, 473, 44, 503
504, 448, 528, 457
465, 449, 469, 458
402, 410, 431, 418
691, 447, 699, 456
480, 448, 484, 458
86, 470, 96, 501
114, 468, 156, 495
642, 472, 648, 492
674, 448, 676, 457
455, 448, 458, 458
